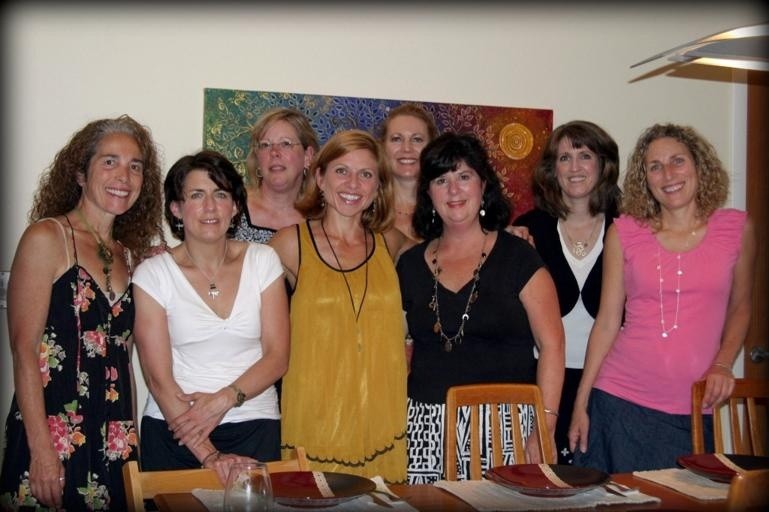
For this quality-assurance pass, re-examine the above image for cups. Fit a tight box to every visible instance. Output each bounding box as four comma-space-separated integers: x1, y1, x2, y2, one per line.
223, 462, 274, 510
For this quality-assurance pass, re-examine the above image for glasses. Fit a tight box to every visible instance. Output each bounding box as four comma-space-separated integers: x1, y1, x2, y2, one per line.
258, 139, 304, 151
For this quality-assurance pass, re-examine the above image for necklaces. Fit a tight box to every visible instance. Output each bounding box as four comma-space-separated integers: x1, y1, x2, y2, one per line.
320, 216, 369, 352
429, 235, 488, 352
183, 239, 230, 301
397, 203, 415, 219
74, 205, 117, 302
655, 214, 704, 339
562, 210, 600, 258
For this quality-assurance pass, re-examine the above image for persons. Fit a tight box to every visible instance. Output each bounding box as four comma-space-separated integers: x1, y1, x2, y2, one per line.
396, 135, 560, 485
381, 103, 438, 243
229, 107, 320, 245
513, 120, 627, 465
137, 128, 536, 486
132, 149, 290, 511
0, 113, 166, 511
566, 122, 756, 471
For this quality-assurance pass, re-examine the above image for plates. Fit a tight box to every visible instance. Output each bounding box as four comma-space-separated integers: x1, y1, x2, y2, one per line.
483, 463, 612, 498
246, 470, 376, 508
676, 453, 769, 482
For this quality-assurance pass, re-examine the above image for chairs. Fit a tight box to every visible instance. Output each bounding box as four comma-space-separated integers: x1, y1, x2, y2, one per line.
724, 468, 769, 510
121, 444, 312, 511
443, 381, 555, 479
688, 373, 768, 457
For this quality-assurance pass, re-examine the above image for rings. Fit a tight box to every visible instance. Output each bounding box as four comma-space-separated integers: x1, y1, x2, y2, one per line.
59, 475, 65, 481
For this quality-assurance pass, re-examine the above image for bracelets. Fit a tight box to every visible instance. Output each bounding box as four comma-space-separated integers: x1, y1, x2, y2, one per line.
229, 384, 246, 408
201, 448, 221, 469
713, 362, 732, 372
542, 406, 561, 417
403, 332, 414, 344
